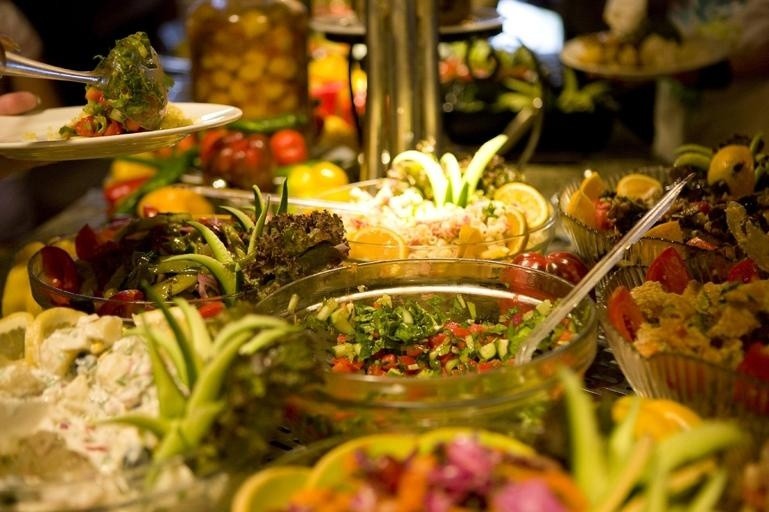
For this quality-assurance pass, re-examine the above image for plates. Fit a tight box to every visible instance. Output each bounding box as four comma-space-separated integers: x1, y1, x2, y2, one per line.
558, 29, 730, 82
0, 100, 243, 163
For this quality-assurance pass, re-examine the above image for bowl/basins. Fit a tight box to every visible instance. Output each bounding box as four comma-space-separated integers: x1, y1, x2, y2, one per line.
245, 257, 599, 443
27, 216, 339, 317
293, 177, 557, 263
595, 264, 769, 444
554, 163, 756, 291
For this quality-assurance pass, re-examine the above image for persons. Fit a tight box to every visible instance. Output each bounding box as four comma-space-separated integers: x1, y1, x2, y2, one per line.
1, 90, 58, 183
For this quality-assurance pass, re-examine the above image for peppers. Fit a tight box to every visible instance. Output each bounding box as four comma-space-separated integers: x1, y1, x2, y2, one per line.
274, 159, 350, 205
502, 251, 594, 329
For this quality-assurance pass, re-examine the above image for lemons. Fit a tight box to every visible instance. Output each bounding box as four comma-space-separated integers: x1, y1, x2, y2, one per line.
0, 149, 214, 385
567, 173, 663, 232
465, 182, 552, 259
345, 228, 406, 279
